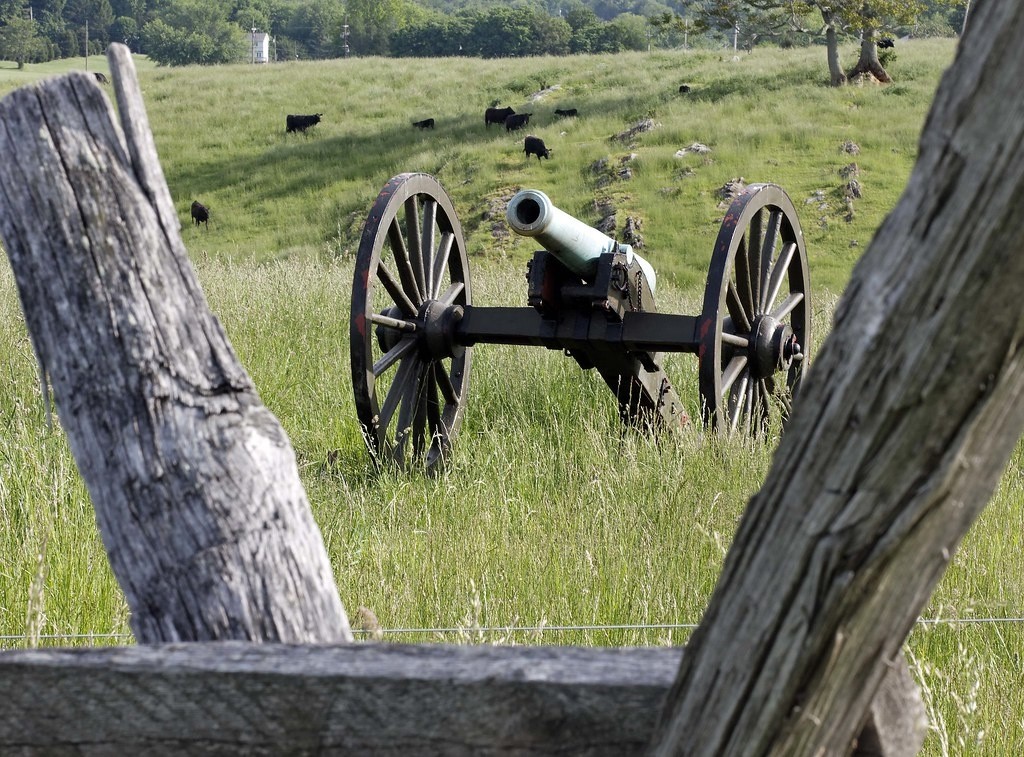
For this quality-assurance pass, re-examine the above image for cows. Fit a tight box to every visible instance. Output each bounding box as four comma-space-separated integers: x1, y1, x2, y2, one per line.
861, 39, 895, 50
412, 118, 435, 131
484, 106, 516, 130
505, 113, 533, 132
554, 109, 579, 118
522, 136, 552, 161
285, 113, 323, 133
191, 200, 210, 230
94, 72, 110, 86
